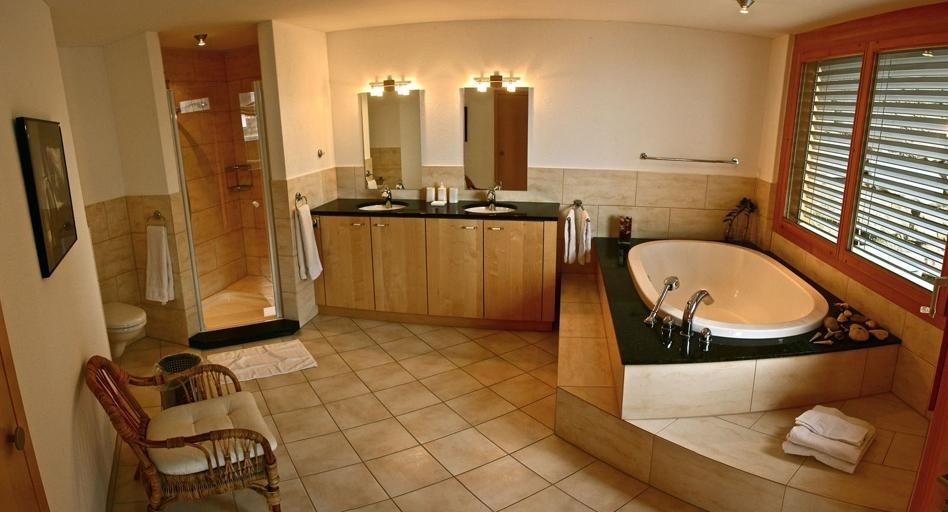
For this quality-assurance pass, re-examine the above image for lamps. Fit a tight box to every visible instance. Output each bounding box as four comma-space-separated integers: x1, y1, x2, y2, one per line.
193, 34, 208, 47
473, 74, 523, 93
736, 0, 754, 16
368, 78, 413, 97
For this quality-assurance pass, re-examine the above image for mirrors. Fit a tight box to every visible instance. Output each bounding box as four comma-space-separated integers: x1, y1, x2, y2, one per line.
462, 87, 528, 191
356, 90, 424, 191
236, 90, 259, 144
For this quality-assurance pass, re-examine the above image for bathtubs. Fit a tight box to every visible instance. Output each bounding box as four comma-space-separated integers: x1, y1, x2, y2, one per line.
626, 238, 830, 342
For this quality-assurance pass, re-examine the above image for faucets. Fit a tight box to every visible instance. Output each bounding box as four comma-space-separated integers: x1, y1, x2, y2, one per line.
486, 189, 496, 202
382, 188, 391, 199
642, 276, 680, 329
395, 182, 406, 189
495, 184, 505, 190
679, 288, 715, 354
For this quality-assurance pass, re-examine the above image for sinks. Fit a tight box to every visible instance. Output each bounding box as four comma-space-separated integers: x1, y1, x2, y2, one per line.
356, 197, 409, 214
461, 200, 519, 214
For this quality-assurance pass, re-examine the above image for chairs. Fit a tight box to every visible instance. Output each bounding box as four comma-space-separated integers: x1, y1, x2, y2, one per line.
83, 353, 282, 512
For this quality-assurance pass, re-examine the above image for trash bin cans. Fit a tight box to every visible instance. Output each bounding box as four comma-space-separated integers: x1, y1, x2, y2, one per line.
154, 353, 203, 411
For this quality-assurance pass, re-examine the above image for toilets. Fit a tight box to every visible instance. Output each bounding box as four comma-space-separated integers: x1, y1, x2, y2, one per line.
102, 301, 147, 361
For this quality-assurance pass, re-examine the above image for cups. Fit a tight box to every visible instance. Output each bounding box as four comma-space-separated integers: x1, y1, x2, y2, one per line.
618, 216, 632, 243
618, 244, 628, 266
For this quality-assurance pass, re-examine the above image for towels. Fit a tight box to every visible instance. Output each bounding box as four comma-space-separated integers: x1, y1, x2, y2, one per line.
577, 209, 592, 266
782, 404, 878, 475
564, 209, 577, 264
205, 340, 318, 385
145, 224, 175, 304
294, 203, 323, 281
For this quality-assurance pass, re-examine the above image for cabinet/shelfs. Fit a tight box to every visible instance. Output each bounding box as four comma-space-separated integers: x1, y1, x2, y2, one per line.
311, 214, 558, 333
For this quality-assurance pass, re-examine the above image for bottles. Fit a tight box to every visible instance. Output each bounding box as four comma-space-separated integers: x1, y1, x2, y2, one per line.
449, 187, 459, 203
426, 187, 435, 203
437, 182, 447, 200
